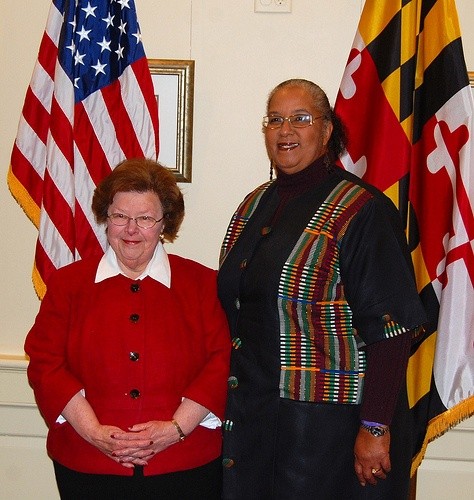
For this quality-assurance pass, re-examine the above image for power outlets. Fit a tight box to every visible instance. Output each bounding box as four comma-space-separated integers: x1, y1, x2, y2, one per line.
254, 0, 292, 13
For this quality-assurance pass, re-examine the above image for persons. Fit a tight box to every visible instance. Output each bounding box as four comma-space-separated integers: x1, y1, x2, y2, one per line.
24, 157, 233, 500
215, 79, 428, 500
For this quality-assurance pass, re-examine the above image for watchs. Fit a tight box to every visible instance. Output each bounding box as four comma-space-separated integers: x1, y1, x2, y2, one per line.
360, 423, 389, 437
170, 419, 186, 442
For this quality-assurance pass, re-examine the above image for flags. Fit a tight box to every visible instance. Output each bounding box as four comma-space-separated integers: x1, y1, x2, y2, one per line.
7, 0, 160, 305
332, 0, 474, 479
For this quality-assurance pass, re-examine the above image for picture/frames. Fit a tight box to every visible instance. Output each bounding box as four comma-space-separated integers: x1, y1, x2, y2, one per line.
148, 59, 195, 184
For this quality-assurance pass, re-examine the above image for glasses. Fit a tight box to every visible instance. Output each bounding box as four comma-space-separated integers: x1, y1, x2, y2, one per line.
262, 113, 326, 129
107, 212, 166, 230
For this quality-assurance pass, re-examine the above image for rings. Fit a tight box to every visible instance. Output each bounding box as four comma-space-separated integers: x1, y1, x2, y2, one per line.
372, 465, 382, 473
